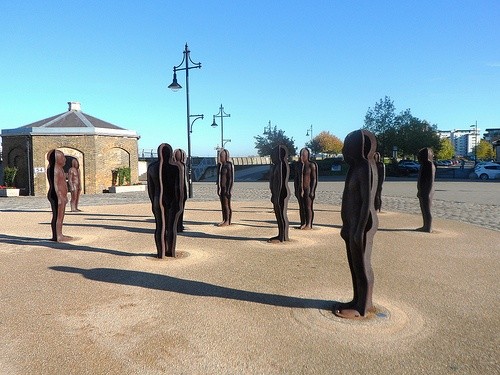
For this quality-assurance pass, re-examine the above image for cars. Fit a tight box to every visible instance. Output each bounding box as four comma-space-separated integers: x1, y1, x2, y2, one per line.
473, 162, 500, 181
397, 159, 460, 171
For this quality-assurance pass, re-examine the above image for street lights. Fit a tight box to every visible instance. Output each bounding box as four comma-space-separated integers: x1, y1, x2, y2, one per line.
305, 124, 313, 141
210, 104, 231, 148
167, 42, 204, 198
470, 121, 477, 163
